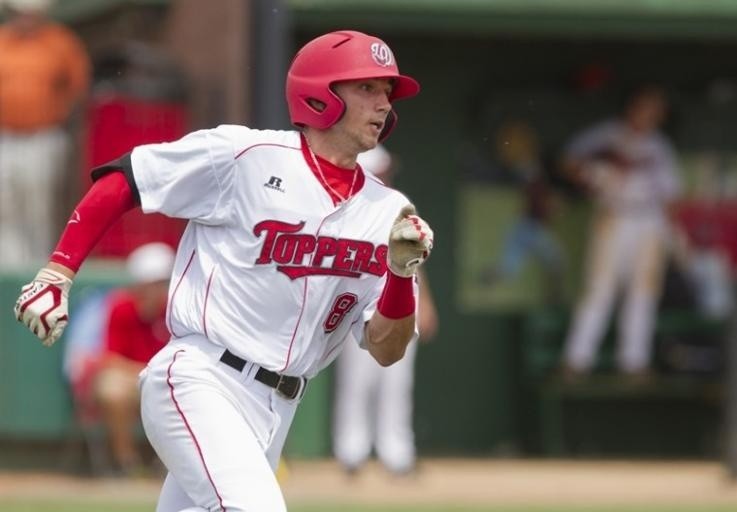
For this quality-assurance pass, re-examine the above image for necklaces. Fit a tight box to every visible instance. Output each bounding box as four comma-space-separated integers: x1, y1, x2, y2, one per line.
302, 131, 358, 203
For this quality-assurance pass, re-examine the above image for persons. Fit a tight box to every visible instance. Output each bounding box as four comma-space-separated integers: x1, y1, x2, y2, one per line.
561, 81, 689, 372
14, 31, 434, 512
332, 145, 439, 474
62, 241, 175, 475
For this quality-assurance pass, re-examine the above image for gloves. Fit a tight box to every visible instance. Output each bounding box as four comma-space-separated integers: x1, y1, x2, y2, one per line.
386, 203, 434, 278
13, 266, 73, 346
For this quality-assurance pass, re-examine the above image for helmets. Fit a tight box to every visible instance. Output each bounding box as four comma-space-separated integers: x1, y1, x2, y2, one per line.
284, 30, 420, 142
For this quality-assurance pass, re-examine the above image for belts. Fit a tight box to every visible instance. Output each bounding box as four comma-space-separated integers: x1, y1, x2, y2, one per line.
219, 349, 308, 400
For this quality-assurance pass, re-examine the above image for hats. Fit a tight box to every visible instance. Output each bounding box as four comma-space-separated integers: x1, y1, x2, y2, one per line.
126, 242, 176, 284
